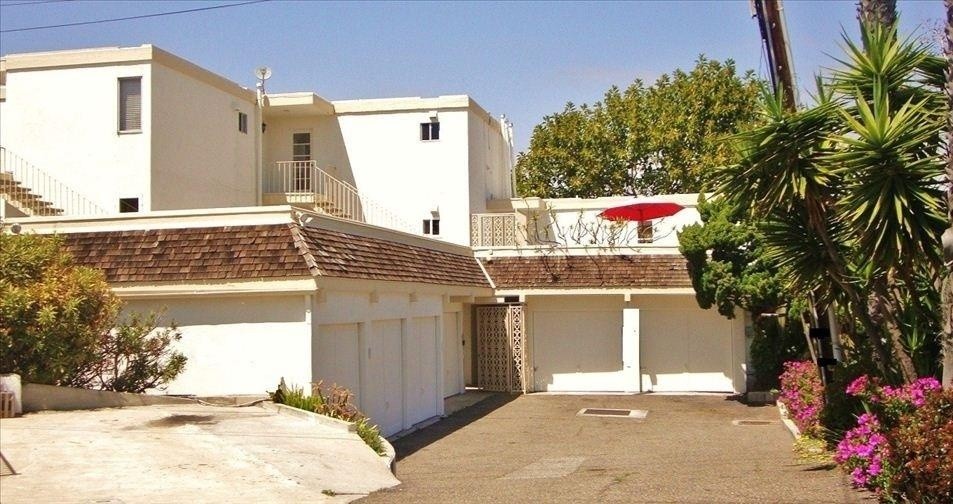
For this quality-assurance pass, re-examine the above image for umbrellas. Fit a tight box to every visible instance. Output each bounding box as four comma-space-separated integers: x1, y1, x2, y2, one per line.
596, 202, 686, 243
